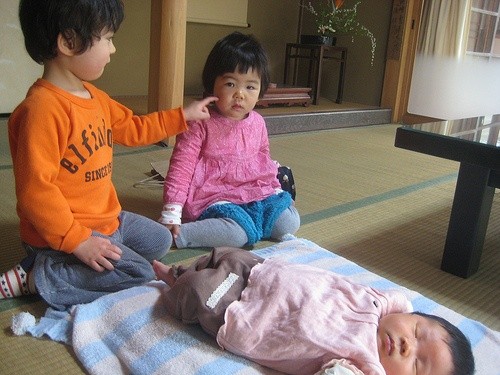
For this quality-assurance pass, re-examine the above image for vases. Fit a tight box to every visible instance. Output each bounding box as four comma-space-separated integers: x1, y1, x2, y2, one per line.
301, 35, 337, 46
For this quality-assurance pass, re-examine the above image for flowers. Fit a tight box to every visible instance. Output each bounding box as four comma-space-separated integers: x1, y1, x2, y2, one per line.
301, 0, 377, 67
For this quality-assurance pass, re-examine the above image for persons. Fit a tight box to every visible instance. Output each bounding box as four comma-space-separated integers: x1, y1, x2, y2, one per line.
0, 0, 219, 309
152, 246, 476, 375
156, 31, 301, 251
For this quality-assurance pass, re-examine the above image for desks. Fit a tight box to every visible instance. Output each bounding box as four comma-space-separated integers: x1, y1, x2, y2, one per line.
284, 42, 348, 106
394, 113, 500, 279
